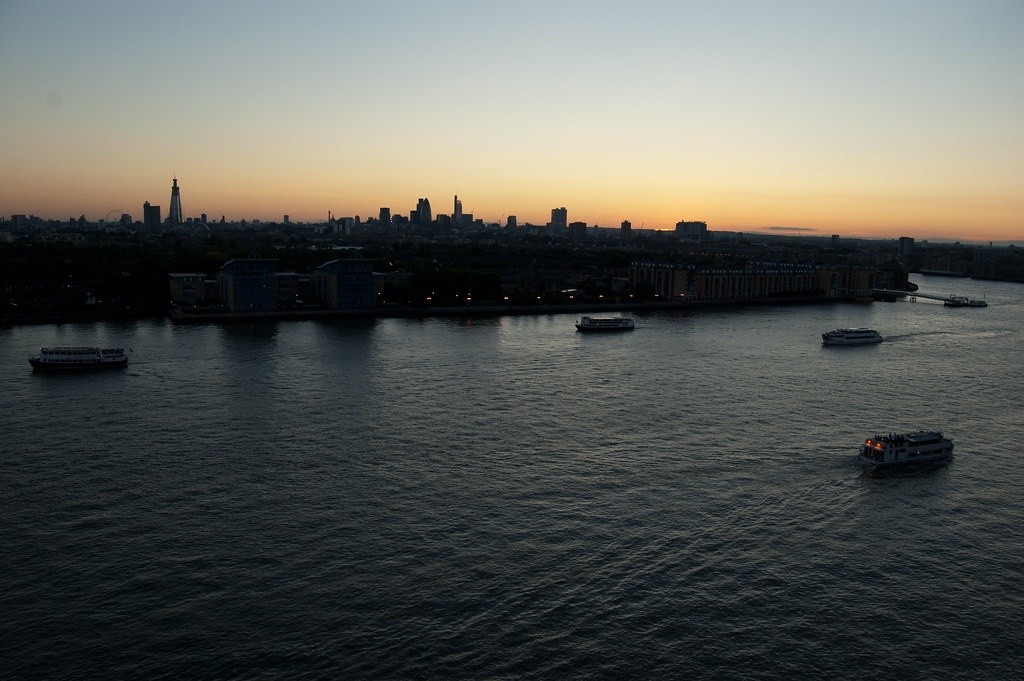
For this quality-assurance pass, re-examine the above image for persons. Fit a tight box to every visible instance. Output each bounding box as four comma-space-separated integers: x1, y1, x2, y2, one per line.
874, 432, 905, 446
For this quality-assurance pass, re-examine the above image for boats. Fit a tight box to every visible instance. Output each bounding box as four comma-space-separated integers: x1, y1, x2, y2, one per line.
821, 326, 882, 347
575, 315, 638, 333
28, 346, 131, 372
858, 431, 954, 471
